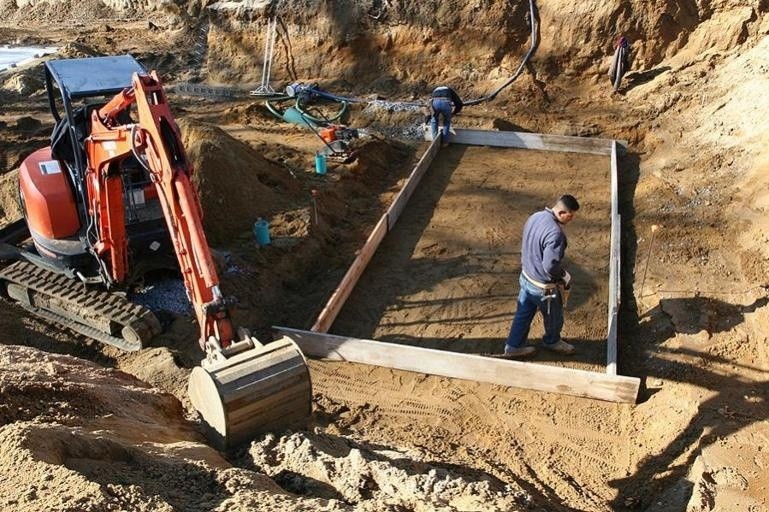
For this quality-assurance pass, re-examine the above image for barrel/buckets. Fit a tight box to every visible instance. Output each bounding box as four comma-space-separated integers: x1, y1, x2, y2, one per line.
316, 154, 327, 175
254, 220, 271, 246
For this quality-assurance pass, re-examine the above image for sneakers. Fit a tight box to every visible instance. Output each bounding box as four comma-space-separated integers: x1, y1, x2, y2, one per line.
505, 343, 534, 357
543, 340, 575, 356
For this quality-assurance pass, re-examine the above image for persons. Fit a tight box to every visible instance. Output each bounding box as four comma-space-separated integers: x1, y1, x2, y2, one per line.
502, 194, 581, 358
426, 84, 465, 149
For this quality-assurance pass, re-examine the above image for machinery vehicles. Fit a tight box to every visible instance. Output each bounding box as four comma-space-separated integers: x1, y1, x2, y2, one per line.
0, 54, 312, 459
